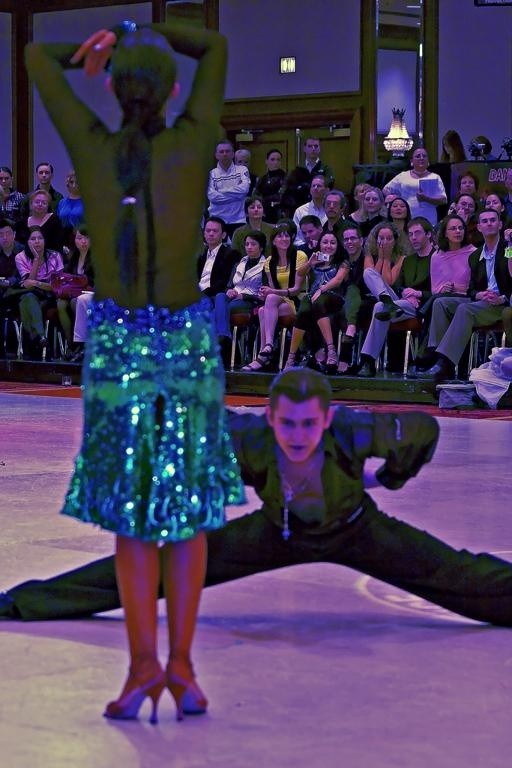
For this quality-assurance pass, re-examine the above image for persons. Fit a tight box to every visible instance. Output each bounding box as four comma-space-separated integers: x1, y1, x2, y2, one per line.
26, 18, 246, 724
0, 368, 512, 630
195, 129, 512, 385
0, 161, 95, 360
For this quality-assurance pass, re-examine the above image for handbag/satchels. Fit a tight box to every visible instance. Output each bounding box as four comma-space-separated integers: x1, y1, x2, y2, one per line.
50, 270, 93, 298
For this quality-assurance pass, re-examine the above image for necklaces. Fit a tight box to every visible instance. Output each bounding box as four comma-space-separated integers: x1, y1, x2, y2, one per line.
271, 445, 323, 541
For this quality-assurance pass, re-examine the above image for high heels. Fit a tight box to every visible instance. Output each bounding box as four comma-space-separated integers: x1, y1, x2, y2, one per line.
103, 673, 165, 724
166, 675, 207, 721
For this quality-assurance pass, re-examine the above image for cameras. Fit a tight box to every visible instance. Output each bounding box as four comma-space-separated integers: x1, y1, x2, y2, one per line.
469, 140, 486, 157
316, 254, 330, 262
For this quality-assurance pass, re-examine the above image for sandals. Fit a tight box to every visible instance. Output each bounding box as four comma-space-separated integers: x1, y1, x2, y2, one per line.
243, 334, 355, 376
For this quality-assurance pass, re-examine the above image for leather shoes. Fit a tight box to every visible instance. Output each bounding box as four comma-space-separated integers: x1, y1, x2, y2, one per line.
357, 363, 376, 377
407, 366, 456, 378
375, 305, 404, 322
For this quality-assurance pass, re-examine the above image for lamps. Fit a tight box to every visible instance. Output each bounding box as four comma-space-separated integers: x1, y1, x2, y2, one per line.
383, 105, 413, 158
